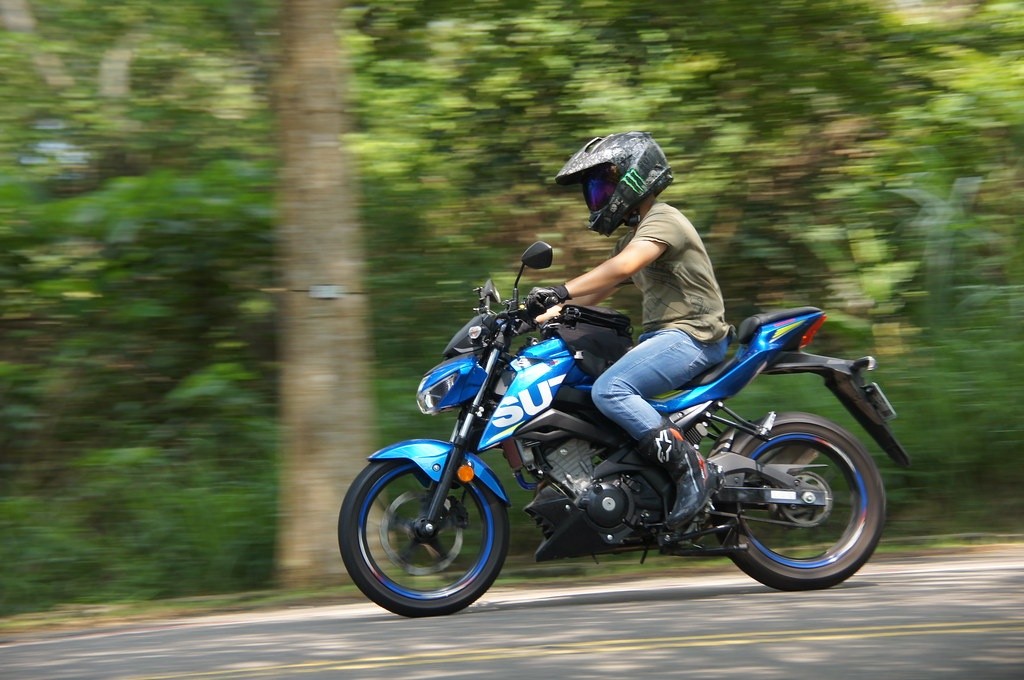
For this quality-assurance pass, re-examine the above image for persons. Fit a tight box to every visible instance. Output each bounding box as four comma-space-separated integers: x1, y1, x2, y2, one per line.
515, 131, 728, 530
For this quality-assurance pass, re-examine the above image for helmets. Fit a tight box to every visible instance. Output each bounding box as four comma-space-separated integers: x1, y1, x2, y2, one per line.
556, 131, 675, 237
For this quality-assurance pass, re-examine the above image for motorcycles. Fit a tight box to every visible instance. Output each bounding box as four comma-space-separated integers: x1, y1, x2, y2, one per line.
337, 239, 909, 620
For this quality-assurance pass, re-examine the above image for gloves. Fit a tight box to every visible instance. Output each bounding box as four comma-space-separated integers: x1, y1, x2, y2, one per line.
527, 284, 573, 319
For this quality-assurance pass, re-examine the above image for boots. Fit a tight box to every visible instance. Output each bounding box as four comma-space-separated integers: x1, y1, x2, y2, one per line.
637, 416, 727, 528
